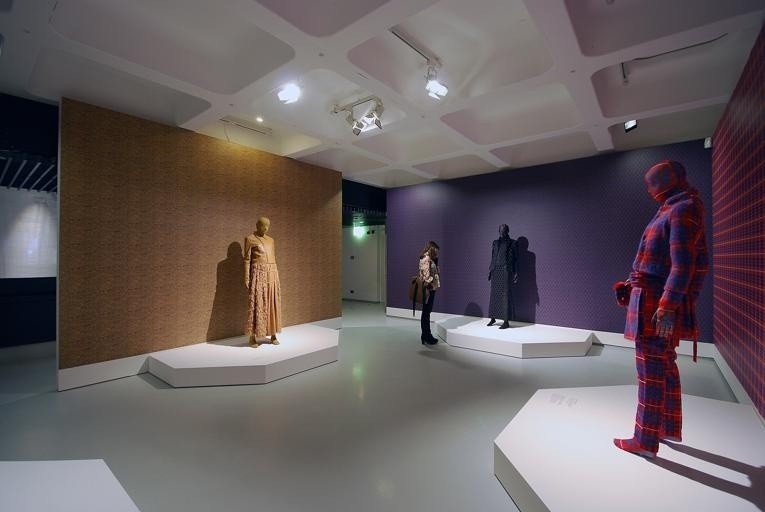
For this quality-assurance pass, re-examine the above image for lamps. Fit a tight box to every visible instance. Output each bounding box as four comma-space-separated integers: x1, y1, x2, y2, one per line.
423, 65, 449, 101
333, 95, 384, 136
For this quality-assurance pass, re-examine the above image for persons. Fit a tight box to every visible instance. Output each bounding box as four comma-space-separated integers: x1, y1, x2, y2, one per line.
243, 217, 281, 348
420, 240, 440, 345
613, 158, 710, 459
486, 224, 519, 329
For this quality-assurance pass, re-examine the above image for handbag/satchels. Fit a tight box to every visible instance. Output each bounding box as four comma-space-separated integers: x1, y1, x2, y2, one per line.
408, 278, 431, 304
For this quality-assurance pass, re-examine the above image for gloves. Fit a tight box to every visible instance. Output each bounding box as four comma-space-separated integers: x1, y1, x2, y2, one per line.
650, 306, 676, 338
613, 281, 630, 308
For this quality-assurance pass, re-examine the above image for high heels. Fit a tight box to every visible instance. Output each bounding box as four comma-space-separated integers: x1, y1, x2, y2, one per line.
421, 333, 438, 345
614, 435, 682, 459
